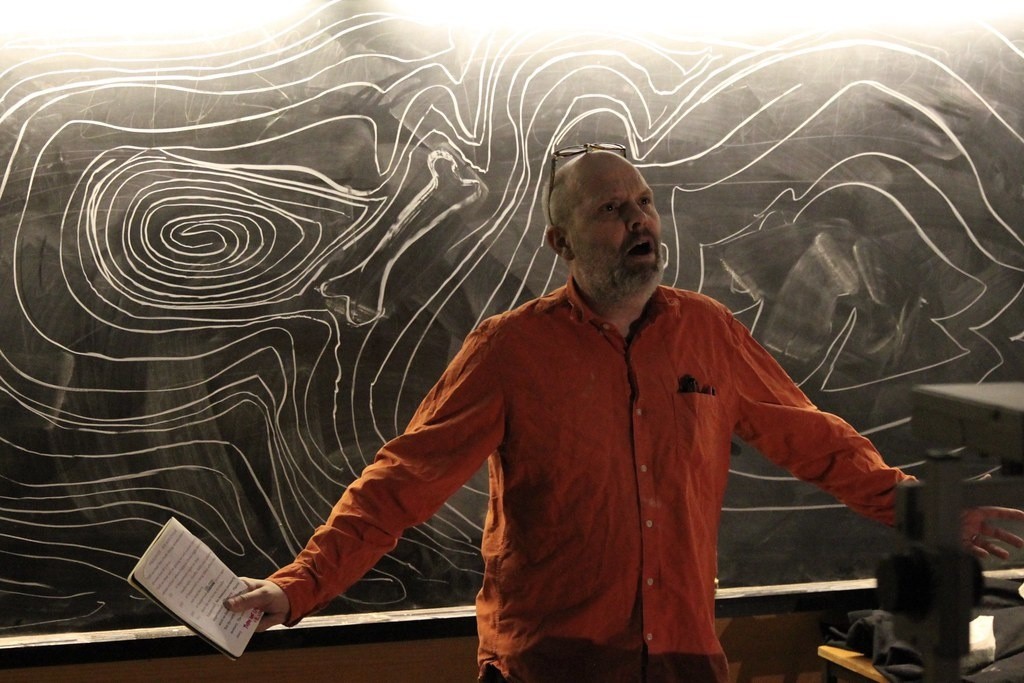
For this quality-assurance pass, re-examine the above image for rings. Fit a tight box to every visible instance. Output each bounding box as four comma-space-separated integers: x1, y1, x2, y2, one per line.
971, 535, 977, 541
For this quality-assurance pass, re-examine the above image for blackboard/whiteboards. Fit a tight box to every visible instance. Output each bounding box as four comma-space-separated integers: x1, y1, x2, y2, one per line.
0, 2, 1023, 669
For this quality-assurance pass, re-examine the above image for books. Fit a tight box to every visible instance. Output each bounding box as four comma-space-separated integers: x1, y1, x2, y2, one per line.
127, 517, 264, 661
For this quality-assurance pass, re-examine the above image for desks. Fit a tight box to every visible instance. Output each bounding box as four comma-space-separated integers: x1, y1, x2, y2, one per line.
817, 643, 892, 683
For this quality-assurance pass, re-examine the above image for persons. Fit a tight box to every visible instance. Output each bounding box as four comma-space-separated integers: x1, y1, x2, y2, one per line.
224, 142, 1024, 683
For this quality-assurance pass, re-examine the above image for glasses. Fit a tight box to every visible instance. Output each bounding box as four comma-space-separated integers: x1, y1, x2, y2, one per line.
547, 142, 626, 225
